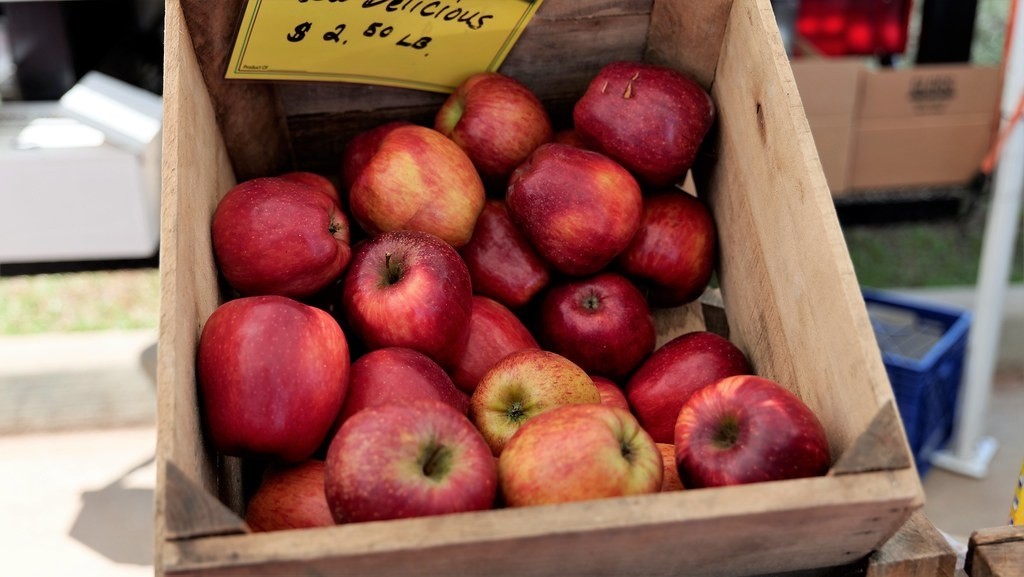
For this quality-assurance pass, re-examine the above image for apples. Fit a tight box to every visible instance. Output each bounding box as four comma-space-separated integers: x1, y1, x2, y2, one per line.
193, 62, 830, 533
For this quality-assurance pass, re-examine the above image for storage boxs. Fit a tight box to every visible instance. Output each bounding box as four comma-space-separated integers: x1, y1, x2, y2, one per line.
855, 286, 970, 482
149, 2, 924, 577
786, 55, 1000, 201
